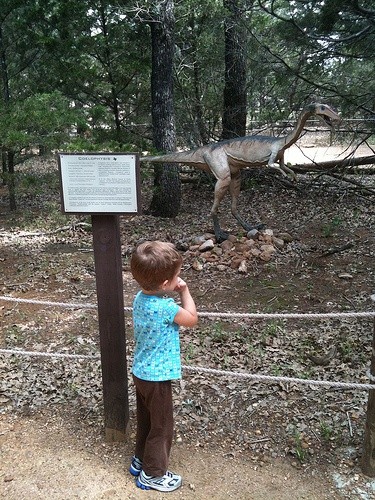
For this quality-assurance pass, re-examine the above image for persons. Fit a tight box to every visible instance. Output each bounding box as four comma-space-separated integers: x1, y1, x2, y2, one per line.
129, 240, 198, 493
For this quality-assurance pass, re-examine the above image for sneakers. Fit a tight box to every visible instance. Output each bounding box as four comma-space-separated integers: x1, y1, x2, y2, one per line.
136, 470, 182, 491
130, 455, 143, 476
